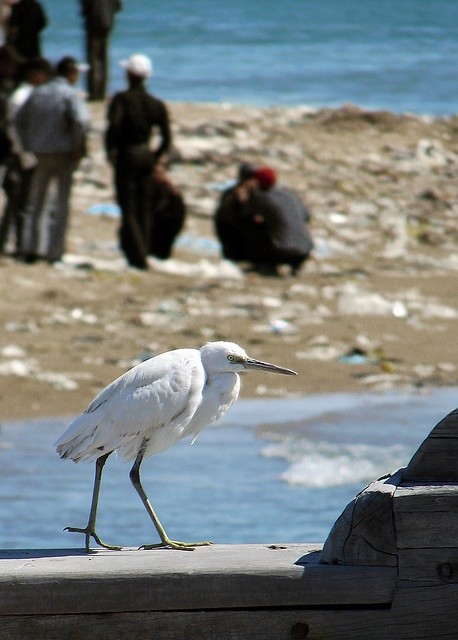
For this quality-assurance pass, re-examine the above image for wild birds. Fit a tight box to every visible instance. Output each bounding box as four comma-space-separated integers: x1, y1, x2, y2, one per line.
51, 340, 298, 553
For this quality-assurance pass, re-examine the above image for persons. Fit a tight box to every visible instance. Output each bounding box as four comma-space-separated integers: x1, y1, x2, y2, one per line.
13, 55, 93, 267
0, 0, 48, 59
1, 41, 33, 172
0, 57, 52, 258
78, 0, 121, 102
212, 163, 266, 262
102, 55, 173, 270
242, 167, 315, 280
145, 157, 187, 261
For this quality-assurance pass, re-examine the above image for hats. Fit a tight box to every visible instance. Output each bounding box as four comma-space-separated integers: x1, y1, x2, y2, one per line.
115, 53, 152, 78
238, 162, 256, 179
253, 167, 275, 185
55, 54, 91, 72
